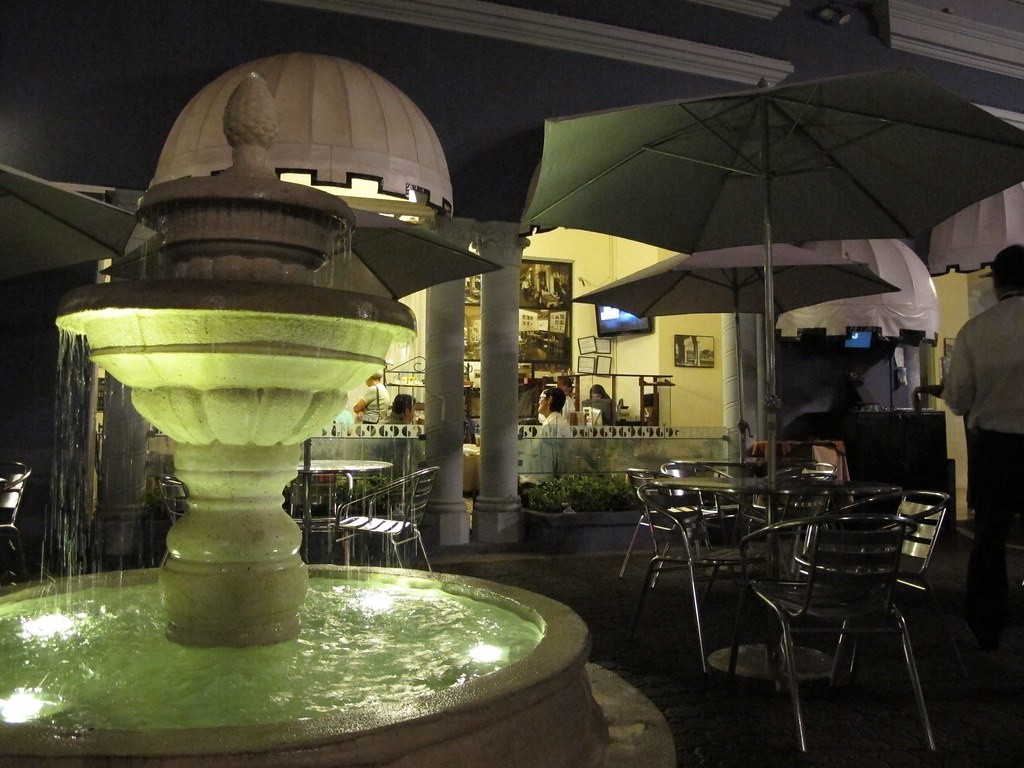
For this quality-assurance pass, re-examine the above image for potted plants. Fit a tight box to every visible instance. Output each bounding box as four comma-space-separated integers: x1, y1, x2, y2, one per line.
520, 475, 650, 555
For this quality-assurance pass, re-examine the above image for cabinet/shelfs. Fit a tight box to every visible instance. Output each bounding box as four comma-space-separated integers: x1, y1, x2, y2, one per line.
383, 357, 473, 423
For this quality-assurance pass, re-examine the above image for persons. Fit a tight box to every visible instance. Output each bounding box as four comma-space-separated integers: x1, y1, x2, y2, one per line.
350, 372, 390, 434
369, 394, 425, 438
538, 376, 575, 426
589, 384, 612, 400
533, 387, 573, 438
940, 244, 1024, 649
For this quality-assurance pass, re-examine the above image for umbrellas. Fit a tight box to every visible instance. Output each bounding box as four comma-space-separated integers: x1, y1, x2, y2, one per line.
571, 243, 901, 594
518, 63, 1023, 680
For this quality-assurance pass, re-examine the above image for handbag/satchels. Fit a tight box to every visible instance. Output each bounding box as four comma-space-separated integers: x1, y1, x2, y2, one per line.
361, 414, 380, 424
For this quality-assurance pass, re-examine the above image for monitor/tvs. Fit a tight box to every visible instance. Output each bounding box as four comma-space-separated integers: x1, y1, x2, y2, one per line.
845, 332, 872, 348
595, 304, 653, 337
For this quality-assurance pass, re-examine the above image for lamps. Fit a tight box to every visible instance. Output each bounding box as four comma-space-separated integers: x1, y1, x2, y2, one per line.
815, 0, 851, 25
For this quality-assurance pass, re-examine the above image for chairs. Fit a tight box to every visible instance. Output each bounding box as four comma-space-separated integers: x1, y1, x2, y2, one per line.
0, 461, 33, 583
619, 461, 953, 755
154, 466, 439, 573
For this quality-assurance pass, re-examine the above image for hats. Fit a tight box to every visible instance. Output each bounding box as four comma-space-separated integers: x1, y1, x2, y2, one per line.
979, 245, 1023, 280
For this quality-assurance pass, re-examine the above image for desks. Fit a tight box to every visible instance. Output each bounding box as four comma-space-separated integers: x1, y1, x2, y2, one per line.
668, 456, 818, 562
650, 478, 904, 691
296, 459, 394, 564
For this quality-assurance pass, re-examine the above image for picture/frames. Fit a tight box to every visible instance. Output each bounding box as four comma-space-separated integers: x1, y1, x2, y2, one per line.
674, 334, 715, 367
